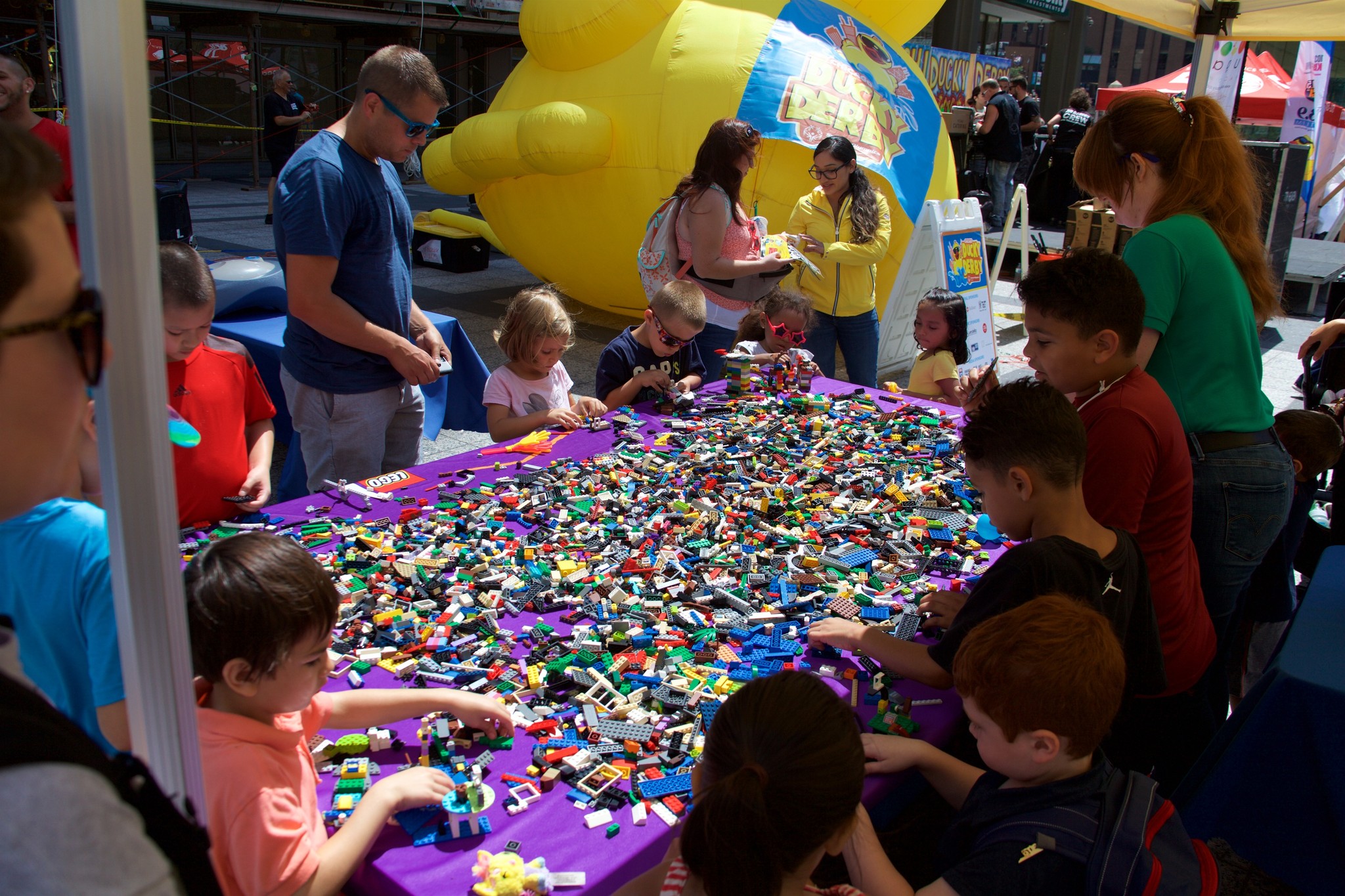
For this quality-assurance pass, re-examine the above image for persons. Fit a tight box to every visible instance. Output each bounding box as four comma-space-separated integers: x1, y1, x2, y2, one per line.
596, 279, 707, 411
183, 534, 516, 896
272, 44, 453, 495
262, 69, 320, 224
610, 671, 867, 896
1298, 319, 1345, 362
1231, 407, 1343, 712
0, 613, 219, 896
881, 287, 968, 408
965, 76, 1094, 233
672, 118, 795, 384
0, 125, 131, 758
806, 378, 1165, 775
159, 240, 276, 530
732, 290, 826, 378
0, 50, 80, 270
1017, 247, 1222, 797
483, 288, 608, 442
842, 595, 1126, 896
785, 135, 892, 389
1072, 91, 1295, 722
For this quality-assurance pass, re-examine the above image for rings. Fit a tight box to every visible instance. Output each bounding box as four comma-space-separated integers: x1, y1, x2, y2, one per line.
815, 241, 818, 244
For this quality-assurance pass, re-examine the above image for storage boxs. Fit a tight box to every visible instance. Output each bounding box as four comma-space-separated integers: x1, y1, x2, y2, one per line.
413, 221, 490, 273
1239, 141, 1311, 309
1061, 199, 1136, 261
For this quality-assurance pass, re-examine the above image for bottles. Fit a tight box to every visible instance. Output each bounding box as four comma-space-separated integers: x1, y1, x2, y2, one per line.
1013, 263, 1021, 283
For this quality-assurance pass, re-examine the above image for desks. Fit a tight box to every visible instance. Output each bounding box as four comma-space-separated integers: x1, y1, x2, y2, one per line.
179, 372, 1031, 896
209, 309, 492, 504
1182, 546, 1345, 895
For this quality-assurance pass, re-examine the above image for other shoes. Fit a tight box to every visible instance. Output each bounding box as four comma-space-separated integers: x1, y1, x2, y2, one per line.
265, 214, 272, 224
988, 225, 1003, 232
1049, 220, 1064, 229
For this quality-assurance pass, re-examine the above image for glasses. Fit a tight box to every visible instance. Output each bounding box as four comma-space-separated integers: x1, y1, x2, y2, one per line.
645, 304, 695, 348
982, 89, 988, 97
364, 88, 440, 138
744, 124, 754, 136
0, 287, 106, 386
1009, 86, 1016, 91
764, 312, 807, 346
286, 80, 294, 84
808, 160, 849, 180
1000, 85, 1008, 89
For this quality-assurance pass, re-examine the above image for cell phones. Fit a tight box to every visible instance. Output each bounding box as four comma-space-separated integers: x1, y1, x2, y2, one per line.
430, 354, 453, 375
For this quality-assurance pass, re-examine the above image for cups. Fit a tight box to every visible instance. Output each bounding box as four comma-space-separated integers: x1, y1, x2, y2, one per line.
750, 215, 769, 237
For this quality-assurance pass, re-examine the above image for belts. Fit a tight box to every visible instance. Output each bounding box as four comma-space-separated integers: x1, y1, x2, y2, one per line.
1187, 426, 1276, 458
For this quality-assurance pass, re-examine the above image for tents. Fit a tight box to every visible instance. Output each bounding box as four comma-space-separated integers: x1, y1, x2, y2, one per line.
1091, 46, 1345, 243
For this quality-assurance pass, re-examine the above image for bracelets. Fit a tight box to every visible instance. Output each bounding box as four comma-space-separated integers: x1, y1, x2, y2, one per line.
1049, 137, 1052, 140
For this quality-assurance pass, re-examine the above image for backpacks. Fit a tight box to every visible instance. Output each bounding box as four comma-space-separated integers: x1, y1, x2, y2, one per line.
638, 180, 732, 305
978, 771, 1226, 896
